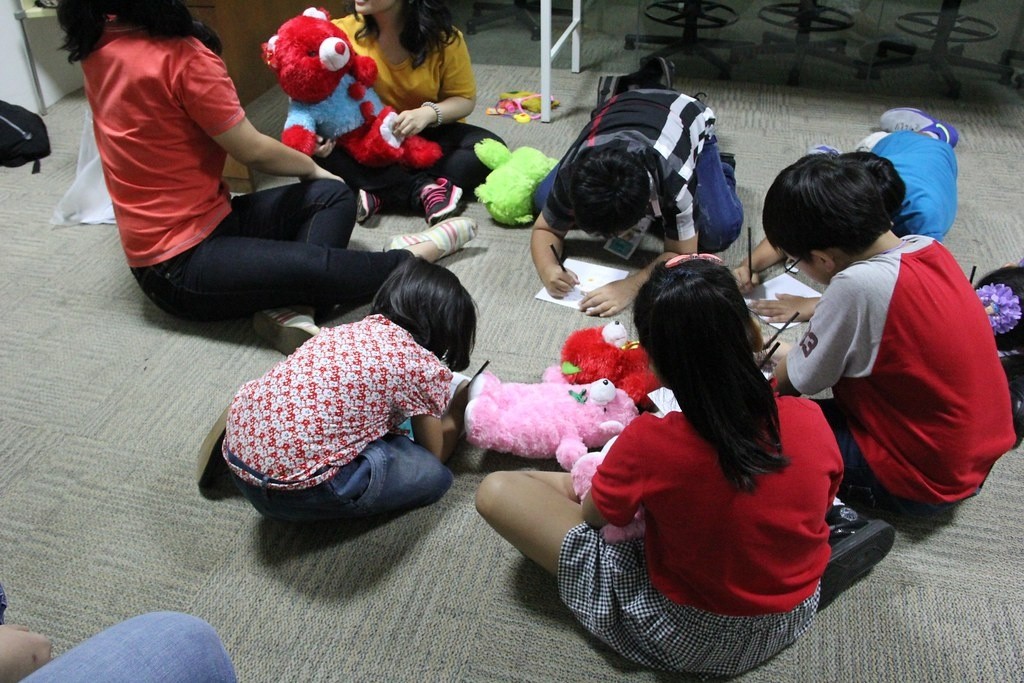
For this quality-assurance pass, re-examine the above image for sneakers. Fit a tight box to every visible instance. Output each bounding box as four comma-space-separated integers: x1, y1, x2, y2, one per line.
880, 107, 958, 148
356, 188, 378, 224
391, 217, 478, 260
420, 178, 465, 224
253, 306, 321, 358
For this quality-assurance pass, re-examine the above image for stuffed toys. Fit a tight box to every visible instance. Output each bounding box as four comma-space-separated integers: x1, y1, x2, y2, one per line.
572, 435, 647, 543
561, 320, 662, 409
473, 137, 559, 225
464, 364, 640, 471
261, 6, 442, 168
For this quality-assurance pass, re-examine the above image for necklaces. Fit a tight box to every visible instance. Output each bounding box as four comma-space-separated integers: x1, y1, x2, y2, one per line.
882, 239, 907, 255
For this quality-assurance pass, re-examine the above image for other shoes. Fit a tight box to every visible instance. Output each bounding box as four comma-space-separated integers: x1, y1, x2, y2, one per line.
720, 151, 735, 170
820, 506, 894, 606
197, 400, 234, 489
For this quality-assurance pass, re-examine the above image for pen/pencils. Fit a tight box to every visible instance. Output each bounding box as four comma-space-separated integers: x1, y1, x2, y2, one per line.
550, 244, 567, 272
969, 265, 976, 283
763, 311, 800, 350
759, 342, 780, 370
748, 226, 752, 278
466, 360, 489, 387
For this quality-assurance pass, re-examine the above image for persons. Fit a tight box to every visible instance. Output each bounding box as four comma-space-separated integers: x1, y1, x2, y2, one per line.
752, 152, 1017, 518
531, 56, 743, 316
195, 258, 475, 522
57, 0, 478, 356
0, 583, 238, 683
732, 105, 958, 321
289, 0, 507, 224
476, 253, 844, 677
973, 258, 1024, 447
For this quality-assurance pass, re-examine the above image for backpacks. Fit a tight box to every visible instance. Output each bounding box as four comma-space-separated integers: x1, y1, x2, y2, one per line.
597, 55, 674, 108
0, 101, 51, 175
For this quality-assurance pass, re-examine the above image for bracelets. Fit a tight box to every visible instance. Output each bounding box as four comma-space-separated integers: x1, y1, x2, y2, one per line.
421, 100, 442, 127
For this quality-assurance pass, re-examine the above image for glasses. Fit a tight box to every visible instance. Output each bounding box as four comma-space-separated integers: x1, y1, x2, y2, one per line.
784, 257, 802, 274
